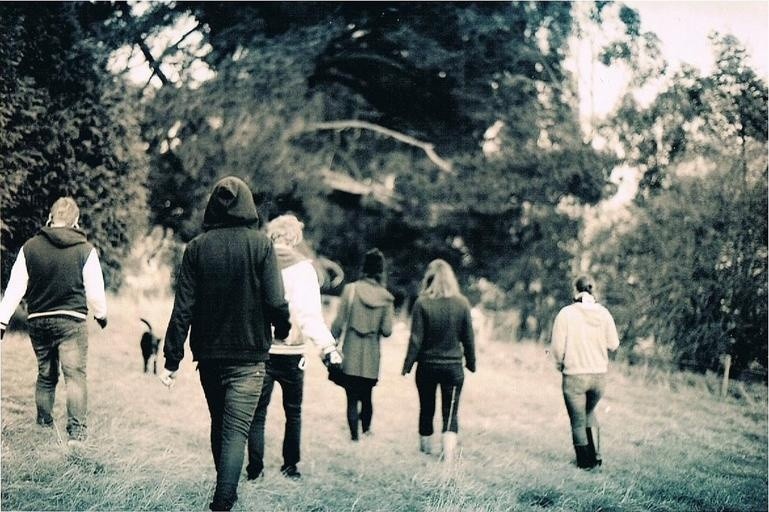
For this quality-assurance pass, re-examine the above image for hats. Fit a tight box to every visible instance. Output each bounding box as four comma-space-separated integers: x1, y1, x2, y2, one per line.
359, 245, 388, 276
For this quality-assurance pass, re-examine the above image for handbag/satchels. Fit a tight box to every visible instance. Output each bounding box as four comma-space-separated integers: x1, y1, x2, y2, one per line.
326, 363, 348, 388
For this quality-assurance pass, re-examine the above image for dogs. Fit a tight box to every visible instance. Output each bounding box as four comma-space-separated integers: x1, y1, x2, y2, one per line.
140, 317, 162, 375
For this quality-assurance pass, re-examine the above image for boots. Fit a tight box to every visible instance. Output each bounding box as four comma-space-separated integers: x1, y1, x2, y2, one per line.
440, 431, 460, 463
588, 425, 604, 468
573, 442, 593, 471
418, 430, 436, 456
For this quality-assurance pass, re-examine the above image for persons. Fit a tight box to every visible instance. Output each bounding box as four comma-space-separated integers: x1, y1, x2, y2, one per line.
0, 196, 107, 450
243, 211, 342, 485
323, 249, 394, 442
552, 276, 620, 468
159, 176, 294, 512
403, 258, 477, 470
